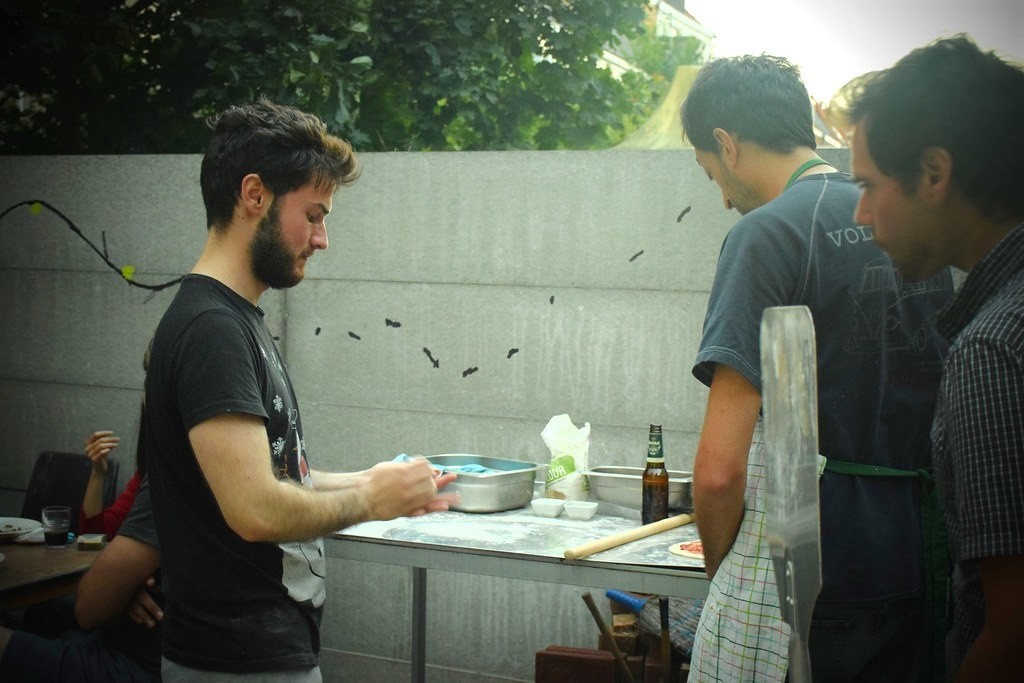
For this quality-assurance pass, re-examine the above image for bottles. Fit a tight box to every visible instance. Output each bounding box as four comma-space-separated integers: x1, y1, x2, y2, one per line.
641, 423, 669, 525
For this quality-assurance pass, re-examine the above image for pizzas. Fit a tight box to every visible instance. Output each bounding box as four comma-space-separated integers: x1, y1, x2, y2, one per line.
669, 539, 704, 560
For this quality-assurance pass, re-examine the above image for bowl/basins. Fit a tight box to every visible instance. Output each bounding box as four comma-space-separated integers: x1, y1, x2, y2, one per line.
530, 497, 565, 518
563, 501, 599, 522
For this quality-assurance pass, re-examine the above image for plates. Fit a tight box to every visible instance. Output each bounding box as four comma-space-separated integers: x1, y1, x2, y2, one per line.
0, 516, 42, 540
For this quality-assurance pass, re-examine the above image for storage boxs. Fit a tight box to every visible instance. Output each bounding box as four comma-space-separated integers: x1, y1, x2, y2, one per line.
77, 533, 107, 549
535, 637, 691, 683
599, 592, 658, 654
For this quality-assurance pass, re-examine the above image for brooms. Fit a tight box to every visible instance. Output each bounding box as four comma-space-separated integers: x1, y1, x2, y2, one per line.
605, 589, 705, 659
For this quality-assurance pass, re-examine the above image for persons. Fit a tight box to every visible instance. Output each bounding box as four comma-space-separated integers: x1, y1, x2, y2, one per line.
142, 93, 462, 682
827, 33, 1024, 683
680, 53, 956, 683
1, 334, 167, 683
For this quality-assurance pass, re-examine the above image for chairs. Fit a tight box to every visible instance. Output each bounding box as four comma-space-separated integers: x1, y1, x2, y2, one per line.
21, 451, 120, 532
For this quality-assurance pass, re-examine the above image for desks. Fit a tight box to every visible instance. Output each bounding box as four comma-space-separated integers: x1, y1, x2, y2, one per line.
0, 538, 112, 612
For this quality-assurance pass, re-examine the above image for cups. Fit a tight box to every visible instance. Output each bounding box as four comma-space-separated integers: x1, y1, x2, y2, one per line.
41, 506, 72, 548
